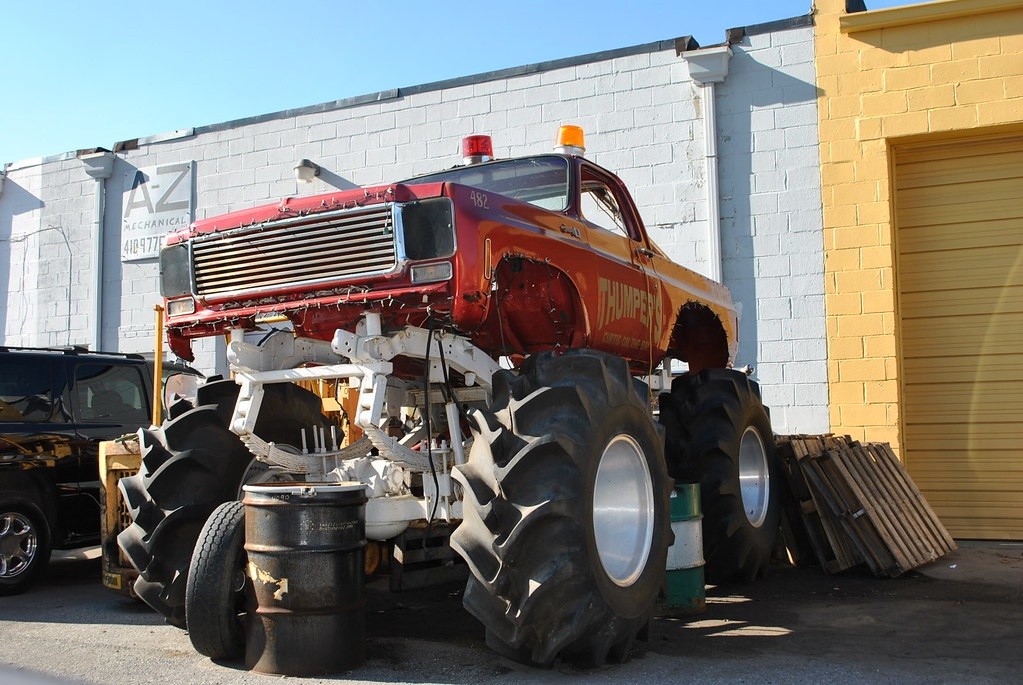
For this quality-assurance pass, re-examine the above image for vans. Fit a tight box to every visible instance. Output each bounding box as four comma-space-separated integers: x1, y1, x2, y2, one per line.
0, 343, 207, 598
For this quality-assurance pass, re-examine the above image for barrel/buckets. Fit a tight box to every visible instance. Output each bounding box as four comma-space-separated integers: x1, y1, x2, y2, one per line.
662, 481, 708, 616
241, 480, 370, 678
